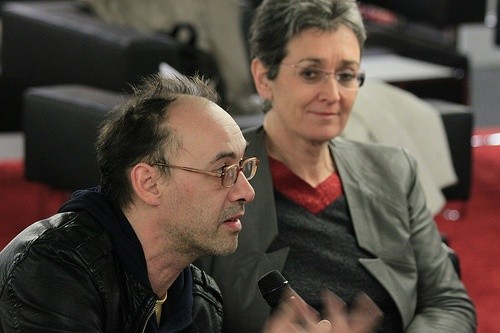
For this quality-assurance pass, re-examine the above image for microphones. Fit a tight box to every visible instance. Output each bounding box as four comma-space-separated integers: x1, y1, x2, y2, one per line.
257, 268, 307, 330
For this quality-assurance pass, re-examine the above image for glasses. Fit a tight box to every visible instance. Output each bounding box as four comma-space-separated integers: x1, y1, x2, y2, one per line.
150, 158, 259, 187
279, 61, 365, 88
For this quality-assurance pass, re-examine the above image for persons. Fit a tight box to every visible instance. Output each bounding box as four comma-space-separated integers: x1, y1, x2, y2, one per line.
241, 0, 457, 217
190, 0, 479, 333
0, 70, 382, 332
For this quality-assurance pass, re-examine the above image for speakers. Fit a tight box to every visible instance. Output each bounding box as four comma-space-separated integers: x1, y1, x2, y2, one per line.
4, 4, 231, 133
26, 83, 138, 193
